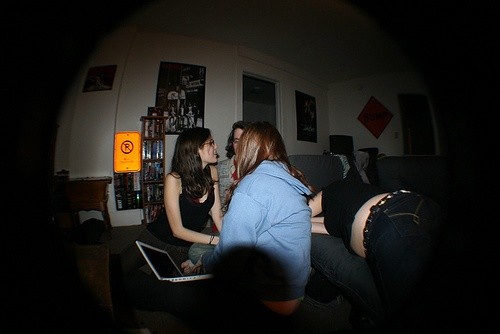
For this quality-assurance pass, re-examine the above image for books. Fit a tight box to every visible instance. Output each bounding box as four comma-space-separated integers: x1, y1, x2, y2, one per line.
143, 120, 165, 225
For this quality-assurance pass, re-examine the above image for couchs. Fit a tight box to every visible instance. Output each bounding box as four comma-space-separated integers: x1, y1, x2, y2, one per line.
188, 155, 500, 333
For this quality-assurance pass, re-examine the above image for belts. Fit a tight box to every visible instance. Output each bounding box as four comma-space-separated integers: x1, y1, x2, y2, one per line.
362, 190, 412, 248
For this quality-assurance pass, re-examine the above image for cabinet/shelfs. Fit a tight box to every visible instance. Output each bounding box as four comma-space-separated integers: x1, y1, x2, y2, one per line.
112, 173, 143, 210
140, 115, 166, 225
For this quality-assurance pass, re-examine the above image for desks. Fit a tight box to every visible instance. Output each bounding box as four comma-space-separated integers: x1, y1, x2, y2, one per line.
45, 176, 110, 237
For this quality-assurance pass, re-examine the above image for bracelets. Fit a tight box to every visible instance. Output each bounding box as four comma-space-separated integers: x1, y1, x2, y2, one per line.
208, 234, 214, 244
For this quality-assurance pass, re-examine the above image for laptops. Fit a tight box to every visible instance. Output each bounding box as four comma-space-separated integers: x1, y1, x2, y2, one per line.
135, 241, 214, 282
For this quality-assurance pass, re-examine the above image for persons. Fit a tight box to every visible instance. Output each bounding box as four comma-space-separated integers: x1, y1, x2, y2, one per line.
110, 127, 222, 284
174, 121, 248, 277
110, 120, 318, 334
309, 177, 444, 334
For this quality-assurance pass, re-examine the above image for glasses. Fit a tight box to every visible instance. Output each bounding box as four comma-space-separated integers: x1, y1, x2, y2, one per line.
203, 140, 214, 146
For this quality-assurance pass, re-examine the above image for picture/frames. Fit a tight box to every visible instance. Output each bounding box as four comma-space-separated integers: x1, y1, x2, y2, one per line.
83, 65, 118, 92
